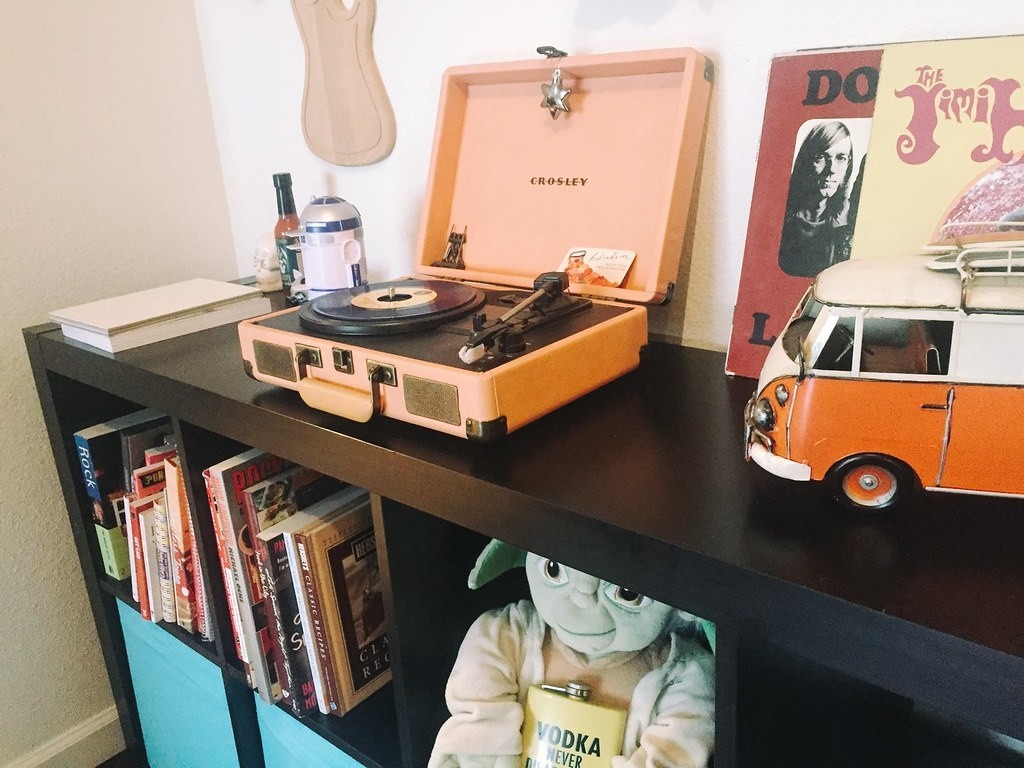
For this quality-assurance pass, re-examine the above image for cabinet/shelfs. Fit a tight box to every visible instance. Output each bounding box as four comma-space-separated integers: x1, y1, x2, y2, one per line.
20, 275, 1024, 768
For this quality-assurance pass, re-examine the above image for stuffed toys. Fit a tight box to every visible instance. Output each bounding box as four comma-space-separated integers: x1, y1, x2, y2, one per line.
426, 535, 714, 768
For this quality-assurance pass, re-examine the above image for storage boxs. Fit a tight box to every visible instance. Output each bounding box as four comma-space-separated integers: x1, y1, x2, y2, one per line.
238, 44, 713, 448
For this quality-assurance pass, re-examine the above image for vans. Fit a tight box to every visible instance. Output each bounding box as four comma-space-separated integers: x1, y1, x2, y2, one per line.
743, 221, 1024, 518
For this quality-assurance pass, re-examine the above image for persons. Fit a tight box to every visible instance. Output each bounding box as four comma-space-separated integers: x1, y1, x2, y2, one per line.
778, 122, 853, 278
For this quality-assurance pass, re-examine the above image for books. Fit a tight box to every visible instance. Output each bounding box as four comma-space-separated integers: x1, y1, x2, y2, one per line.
71, 407, 392, 720
43, 278, 273, 355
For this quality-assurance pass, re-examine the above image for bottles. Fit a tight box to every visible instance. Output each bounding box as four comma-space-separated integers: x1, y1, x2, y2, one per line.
273, 173, 301, 302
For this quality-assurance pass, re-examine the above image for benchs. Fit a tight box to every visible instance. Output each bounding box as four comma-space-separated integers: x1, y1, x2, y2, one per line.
861, 320, 942, 375
